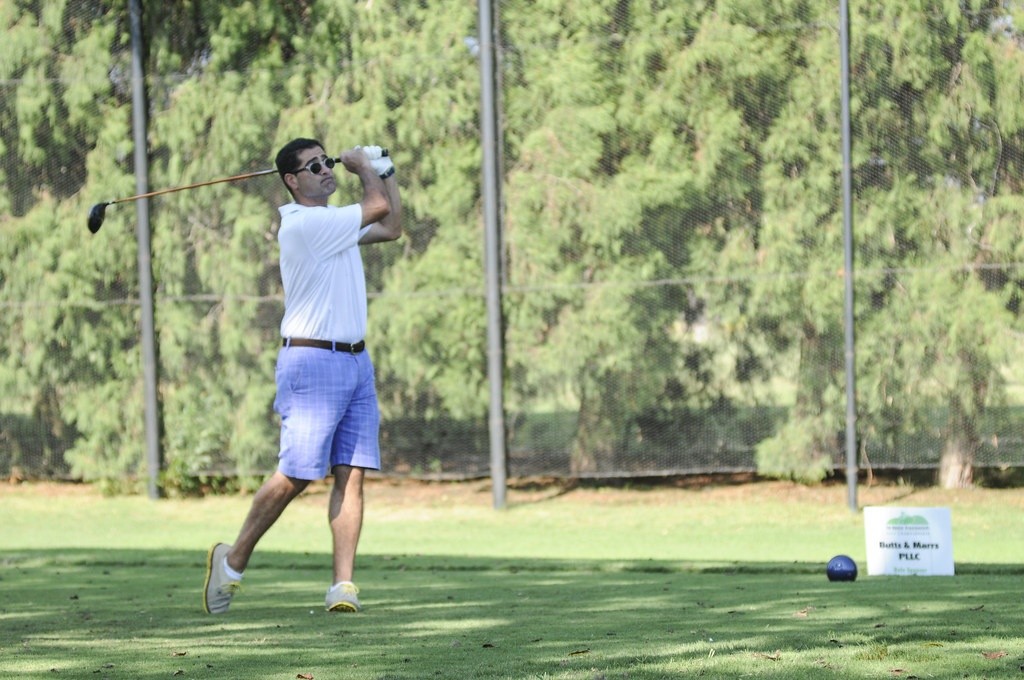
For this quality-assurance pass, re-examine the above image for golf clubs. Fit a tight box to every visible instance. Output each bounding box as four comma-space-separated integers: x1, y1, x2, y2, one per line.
88, 149, 388, 234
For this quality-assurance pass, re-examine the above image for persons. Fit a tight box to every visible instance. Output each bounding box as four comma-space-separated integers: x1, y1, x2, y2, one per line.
202, 137, 402, 614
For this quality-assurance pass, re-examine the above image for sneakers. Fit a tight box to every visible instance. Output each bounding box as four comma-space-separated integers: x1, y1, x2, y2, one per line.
324, 582, 361, 612
202, 541, 250, 618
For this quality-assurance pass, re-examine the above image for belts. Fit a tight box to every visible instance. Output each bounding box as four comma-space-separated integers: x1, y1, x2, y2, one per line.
282, 336, 365, 355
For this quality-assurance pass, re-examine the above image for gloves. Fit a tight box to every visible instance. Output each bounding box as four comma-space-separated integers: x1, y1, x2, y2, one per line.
354, 145, 395, 179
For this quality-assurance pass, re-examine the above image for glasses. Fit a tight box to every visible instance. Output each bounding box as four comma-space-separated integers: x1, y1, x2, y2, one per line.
294, 157, 336, 175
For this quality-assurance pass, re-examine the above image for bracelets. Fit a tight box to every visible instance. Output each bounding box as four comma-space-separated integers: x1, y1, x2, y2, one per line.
380, 168, 394, 179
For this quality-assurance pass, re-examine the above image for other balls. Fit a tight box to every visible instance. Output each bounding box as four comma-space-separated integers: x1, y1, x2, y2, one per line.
826, 555, 858, 582
